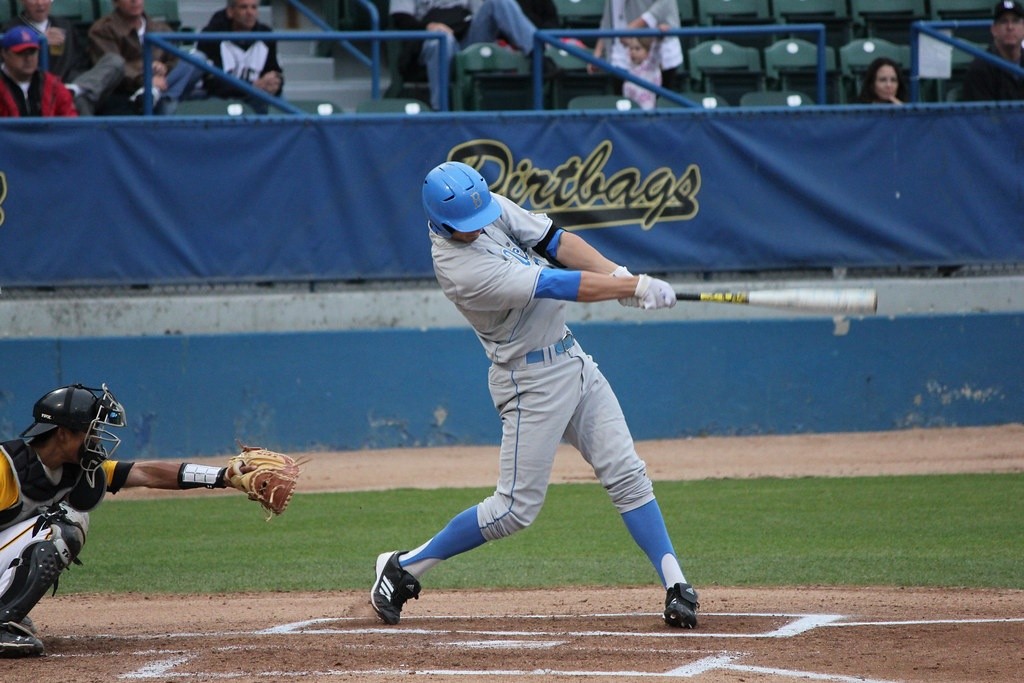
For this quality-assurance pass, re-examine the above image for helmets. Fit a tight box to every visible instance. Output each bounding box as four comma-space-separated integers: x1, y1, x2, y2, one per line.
421, 159, 503, 239
19, 385, 101, 436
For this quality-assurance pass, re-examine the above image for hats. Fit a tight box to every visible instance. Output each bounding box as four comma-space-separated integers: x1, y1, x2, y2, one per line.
0, 25, 41, 54
994, 0, 1023, 23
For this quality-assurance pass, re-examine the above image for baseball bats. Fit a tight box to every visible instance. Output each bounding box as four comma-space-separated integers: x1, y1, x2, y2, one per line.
674, 287, 878, 318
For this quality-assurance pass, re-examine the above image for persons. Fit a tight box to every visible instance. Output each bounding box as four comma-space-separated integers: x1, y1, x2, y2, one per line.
0, 382, 314, 659
369, 162, 700, 630
959, 0, 1024, 102
0, 0, 689, 116
857, 56, 964, 272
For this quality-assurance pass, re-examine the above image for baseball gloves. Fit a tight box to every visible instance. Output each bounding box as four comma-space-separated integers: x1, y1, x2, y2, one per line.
226, 443, 300, 517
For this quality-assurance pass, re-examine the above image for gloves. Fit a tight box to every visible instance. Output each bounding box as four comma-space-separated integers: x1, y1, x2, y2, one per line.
633, 272, 676, 311
609, 266, 645, 310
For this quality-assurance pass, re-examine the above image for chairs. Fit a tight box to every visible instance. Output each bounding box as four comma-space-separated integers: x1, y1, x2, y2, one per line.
0, 0, 1024, 115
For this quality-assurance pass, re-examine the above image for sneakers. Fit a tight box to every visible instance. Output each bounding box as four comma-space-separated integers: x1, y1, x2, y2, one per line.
370, 550, 422, 625
663, 583, 700, 629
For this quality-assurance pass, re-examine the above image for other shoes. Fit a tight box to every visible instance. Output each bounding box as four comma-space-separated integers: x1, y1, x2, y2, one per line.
126, 85, 161, 110
